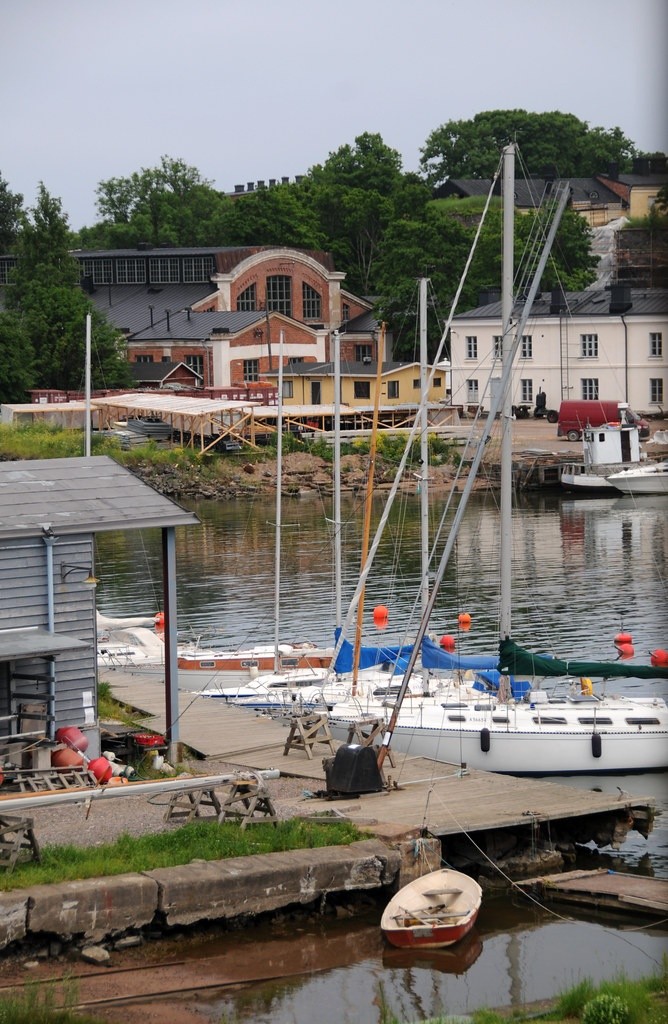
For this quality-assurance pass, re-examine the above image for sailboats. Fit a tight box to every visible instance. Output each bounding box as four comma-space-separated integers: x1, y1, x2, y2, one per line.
96, 143, 668, 775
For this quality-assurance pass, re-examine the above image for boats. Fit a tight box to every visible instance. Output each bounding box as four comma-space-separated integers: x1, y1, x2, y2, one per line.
380, 867, 483, 949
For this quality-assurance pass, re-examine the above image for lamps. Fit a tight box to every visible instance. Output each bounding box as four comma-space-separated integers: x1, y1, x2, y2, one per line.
60, 561, 100, 590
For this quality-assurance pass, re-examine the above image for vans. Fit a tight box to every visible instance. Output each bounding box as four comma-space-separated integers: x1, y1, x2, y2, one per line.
557, 400, 651, 442
